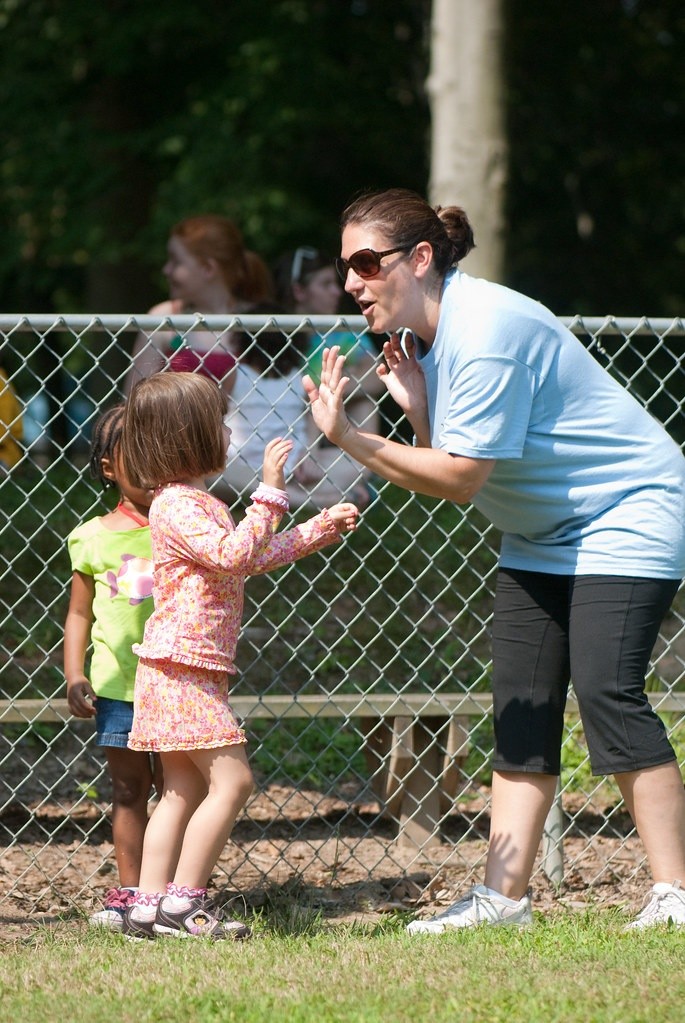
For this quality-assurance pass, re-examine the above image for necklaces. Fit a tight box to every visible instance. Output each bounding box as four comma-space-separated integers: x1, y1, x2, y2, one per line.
117, 501, 151, 529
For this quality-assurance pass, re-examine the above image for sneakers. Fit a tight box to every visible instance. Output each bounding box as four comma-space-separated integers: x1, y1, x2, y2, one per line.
402, 885, 532, 946
625, 886, 682, 937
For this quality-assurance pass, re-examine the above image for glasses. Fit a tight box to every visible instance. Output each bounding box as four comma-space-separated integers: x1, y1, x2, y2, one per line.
335, 246, 407, 280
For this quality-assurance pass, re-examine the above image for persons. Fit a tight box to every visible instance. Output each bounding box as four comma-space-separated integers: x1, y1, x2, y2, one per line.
208, 298, 372, 509
119, 372, 357, 947
120, 213, 277, 399
300, 191, 685, 939
64, 405, 160, 943
274, 242, 391, 488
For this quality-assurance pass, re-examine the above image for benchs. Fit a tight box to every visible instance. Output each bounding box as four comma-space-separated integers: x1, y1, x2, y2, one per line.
3, 687, 683, 857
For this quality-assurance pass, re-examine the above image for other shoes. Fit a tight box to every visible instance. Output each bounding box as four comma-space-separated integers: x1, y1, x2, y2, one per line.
123, 892, 251, 949
93, 889, 132, 929
